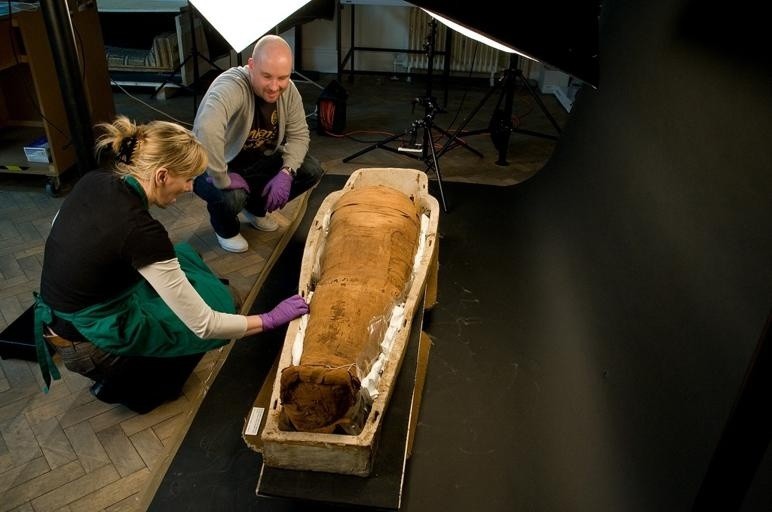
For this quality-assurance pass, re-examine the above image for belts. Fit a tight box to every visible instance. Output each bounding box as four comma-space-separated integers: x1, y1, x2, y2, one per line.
42, 334, 80, 347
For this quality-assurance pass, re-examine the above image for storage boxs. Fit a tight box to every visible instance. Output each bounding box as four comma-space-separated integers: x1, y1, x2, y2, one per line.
538, 64, 562, 94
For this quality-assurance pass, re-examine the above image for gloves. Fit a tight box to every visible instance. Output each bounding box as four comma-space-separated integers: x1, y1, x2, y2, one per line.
262, 171, 294, 213
259, 294, 310, 332
206, 173, 249, 193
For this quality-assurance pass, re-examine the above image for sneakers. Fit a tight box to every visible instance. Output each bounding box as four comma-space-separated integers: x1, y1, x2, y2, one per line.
215, 231, 249, 253
242, 207, 279, 232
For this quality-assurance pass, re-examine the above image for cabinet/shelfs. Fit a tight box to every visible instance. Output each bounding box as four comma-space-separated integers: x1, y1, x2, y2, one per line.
66, 0, 117, 129
0, 4, 77, 197
96, 0, 211, 101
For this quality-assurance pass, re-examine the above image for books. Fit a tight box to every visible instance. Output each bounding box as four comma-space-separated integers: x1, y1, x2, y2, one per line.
152, 33, 180, 68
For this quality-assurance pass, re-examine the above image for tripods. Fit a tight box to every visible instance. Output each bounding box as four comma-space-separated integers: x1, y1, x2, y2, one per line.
150, 3, 225, 122
424, 54, 561, 173
343, 23, 483, 213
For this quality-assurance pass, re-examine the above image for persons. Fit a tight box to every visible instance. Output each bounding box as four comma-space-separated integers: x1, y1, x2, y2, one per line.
189, 34, 324, 253
36, 117, 308, 414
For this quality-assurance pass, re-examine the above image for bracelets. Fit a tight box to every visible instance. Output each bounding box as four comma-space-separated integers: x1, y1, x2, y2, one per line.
285, 166, 296, 179
